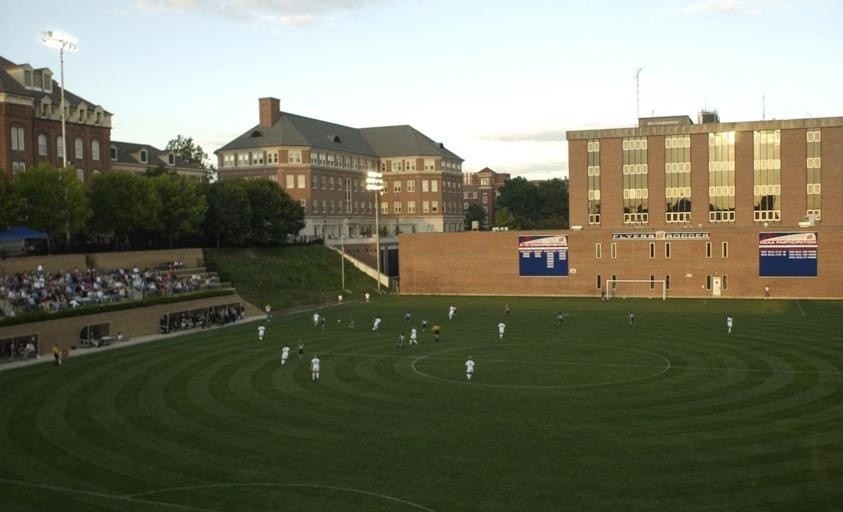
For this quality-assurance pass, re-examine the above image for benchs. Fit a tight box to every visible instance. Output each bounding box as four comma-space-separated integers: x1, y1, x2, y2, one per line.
0, 263, 243, 364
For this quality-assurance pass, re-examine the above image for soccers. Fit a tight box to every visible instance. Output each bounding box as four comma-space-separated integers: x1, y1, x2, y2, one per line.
337, 319, 341, 323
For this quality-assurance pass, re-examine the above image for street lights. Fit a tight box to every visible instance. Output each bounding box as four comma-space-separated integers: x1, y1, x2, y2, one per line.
33, 24, 83, 248
364, 168, 388, 292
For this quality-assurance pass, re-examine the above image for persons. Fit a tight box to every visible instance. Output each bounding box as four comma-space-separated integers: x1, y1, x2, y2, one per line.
497, 320, 506, 340
465, 356, 476, 380
726, 314, 734, 335
627, 311, 635, 328
557, 311, 564, 331
764, 284, 770, 297
504, 304, 511, 318
448, 303, 457, 320
